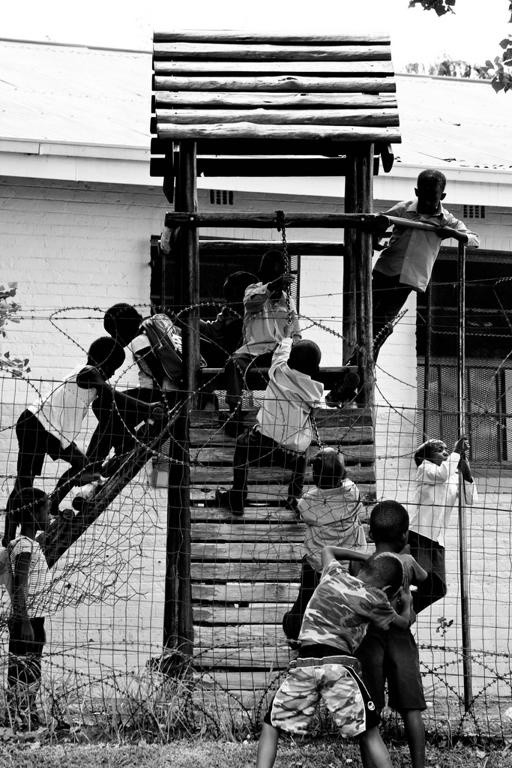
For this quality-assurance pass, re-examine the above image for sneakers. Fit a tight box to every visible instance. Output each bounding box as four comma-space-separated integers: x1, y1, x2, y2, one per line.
214, 485, 242, 516
324, 390, 341, 408
281, 610, 300, 651
218, 412, 244, 438
334, 371, 360, 398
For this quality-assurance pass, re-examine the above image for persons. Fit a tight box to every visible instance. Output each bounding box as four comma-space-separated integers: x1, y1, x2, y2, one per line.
1, 304, 207, 548
254, 448, 427, 768
213, 247, 325, 517
404, 439, 479, 616
324, 167, 480, 408
0, 487, 52, 740
